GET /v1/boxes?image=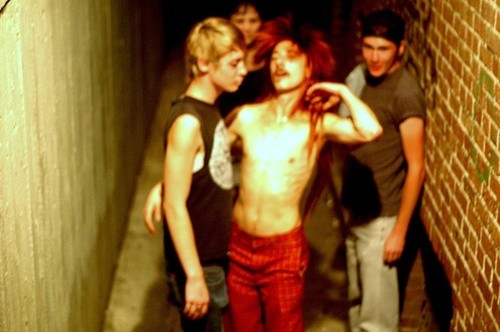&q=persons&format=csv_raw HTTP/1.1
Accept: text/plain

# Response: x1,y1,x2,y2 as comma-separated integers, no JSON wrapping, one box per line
332,8,430,332
214,0,267,119
141,13,384,332
159,17,250,332
